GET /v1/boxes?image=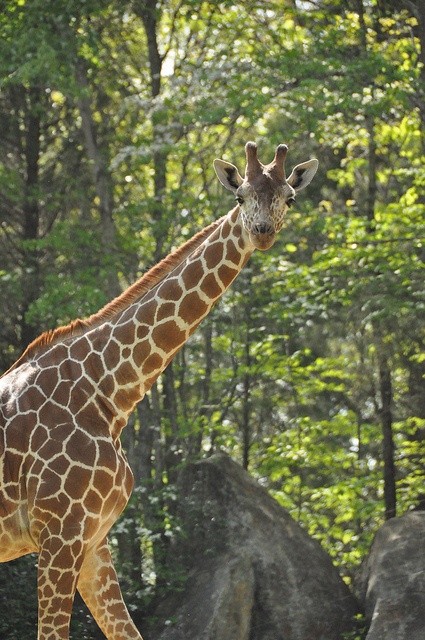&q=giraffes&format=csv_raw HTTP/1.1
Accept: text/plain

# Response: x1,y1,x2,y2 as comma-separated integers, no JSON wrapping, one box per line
1,139,321,640
